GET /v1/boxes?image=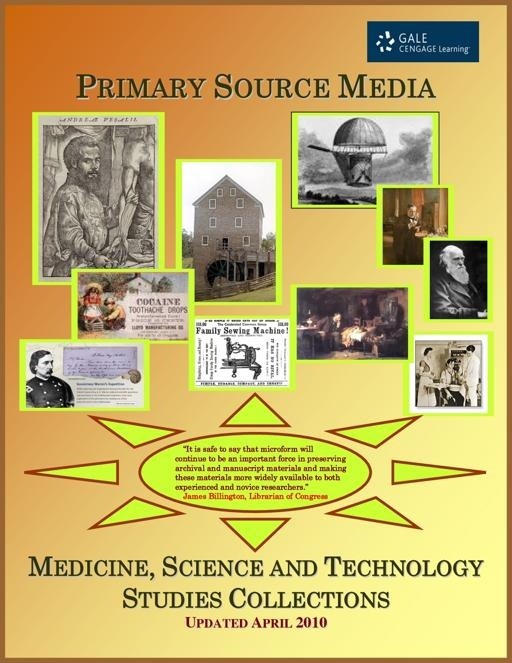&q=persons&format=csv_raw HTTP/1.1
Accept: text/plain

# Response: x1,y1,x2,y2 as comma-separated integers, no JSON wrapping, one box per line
80,282,126,332
430,245,488,318
416,344,481,407
26,350,75,408
392,204,418,264
43,135,120,276
109,124,155,265
315,313,342,352
